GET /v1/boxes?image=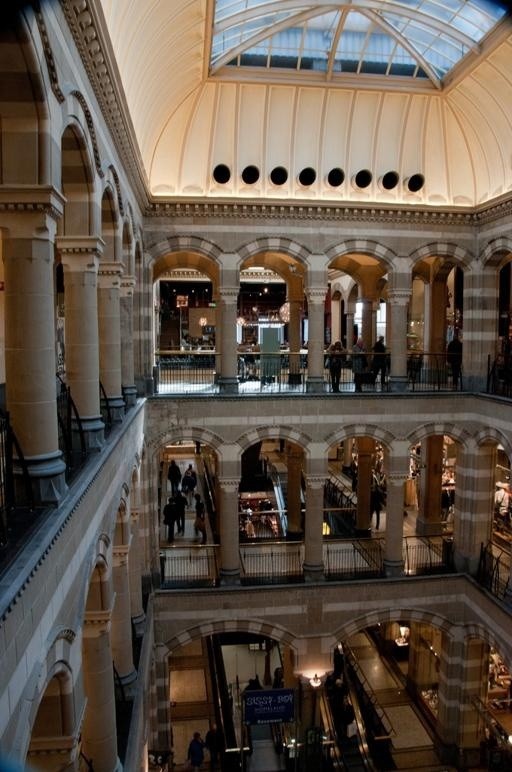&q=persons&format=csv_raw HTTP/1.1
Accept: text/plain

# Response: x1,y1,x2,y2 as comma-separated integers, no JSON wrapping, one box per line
351,339,368,392
161,457,209,546
327,341,348,393
187,732,205,771
370,335,388,390
205,721,225,772
